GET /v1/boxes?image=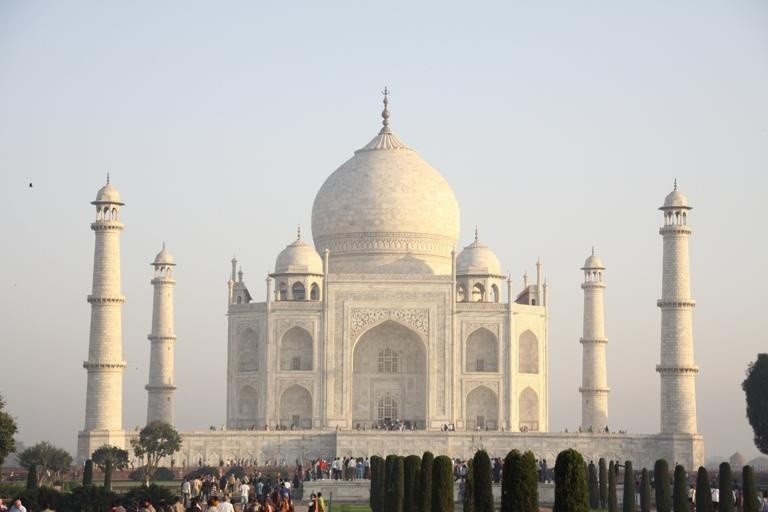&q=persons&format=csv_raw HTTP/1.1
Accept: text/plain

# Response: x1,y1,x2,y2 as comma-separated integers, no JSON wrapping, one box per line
355,416,419,432
687,484,767,512
485,425,488,432
0,489,295,512
170,453,370,490
558,423,627,434
208,421,297,433
314,493,326,512
473,425,482,432
307,494,317,512
501,426,504,432
613,460,624,482
587,460,594,477
451,453,549,484
519,424,528,433
439,423,454,432
334,423,339,431
